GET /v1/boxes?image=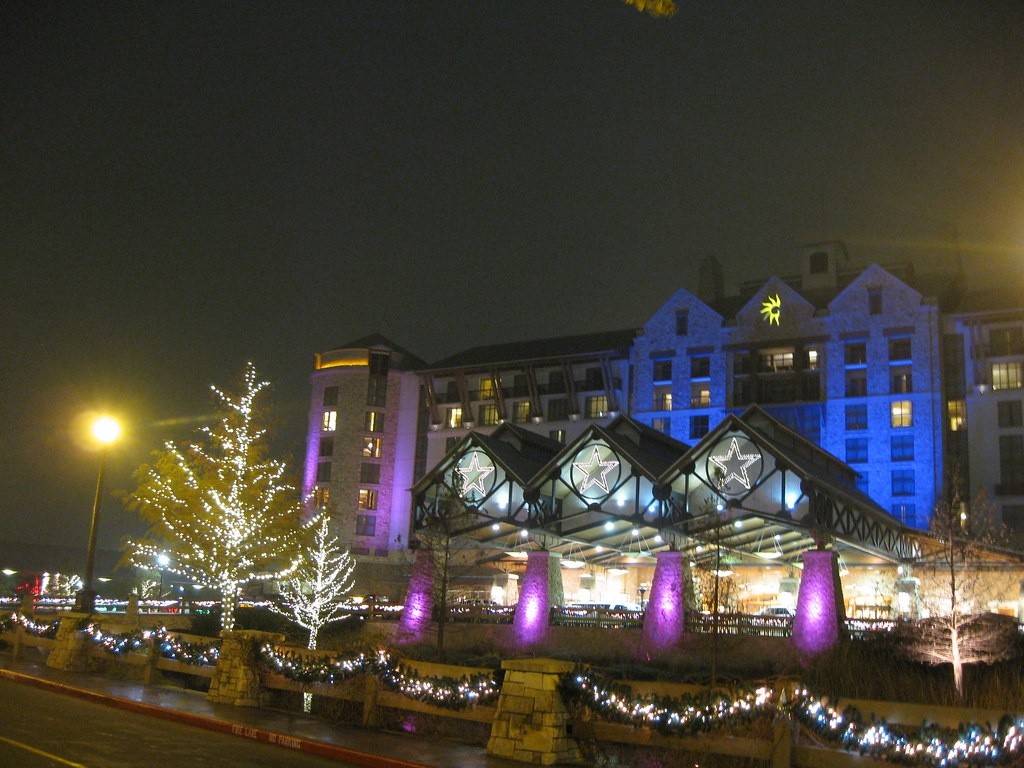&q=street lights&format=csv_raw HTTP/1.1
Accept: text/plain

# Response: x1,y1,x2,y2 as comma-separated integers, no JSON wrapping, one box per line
68,412,119,613
157,554,170,613
